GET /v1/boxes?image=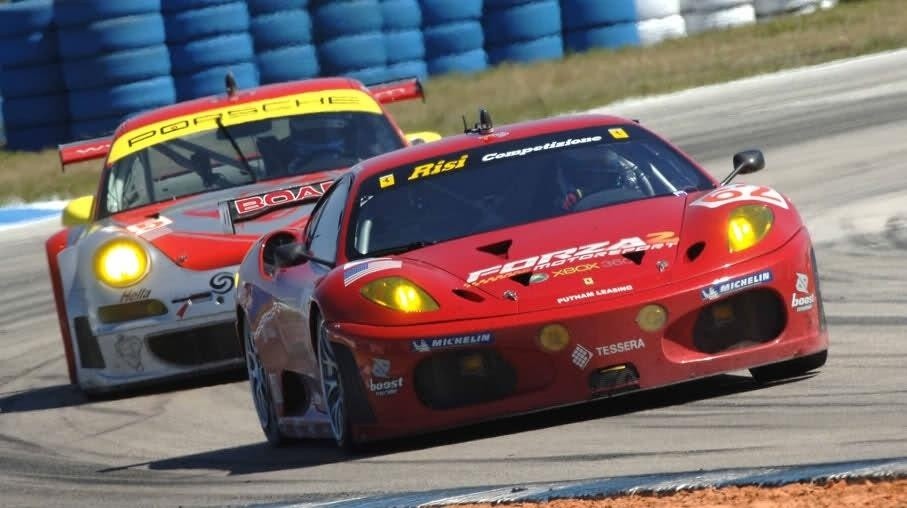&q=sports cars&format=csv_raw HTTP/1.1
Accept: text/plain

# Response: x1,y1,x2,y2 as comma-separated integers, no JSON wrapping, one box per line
234,108,826,459
42,70,440,400
